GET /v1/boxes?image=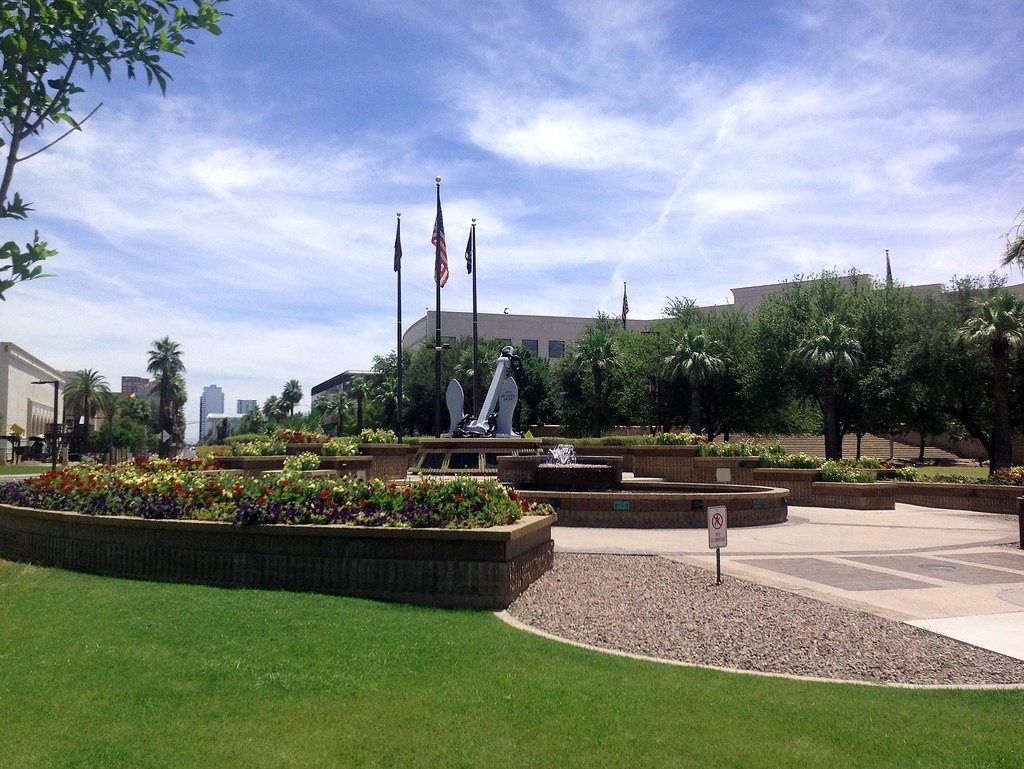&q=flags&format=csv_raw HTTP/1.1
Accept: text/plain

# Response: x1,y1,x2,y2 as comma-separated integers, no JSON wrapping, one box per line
431,202,449,288
622,296,629,324
394,222,402,272
465,227,472,274
